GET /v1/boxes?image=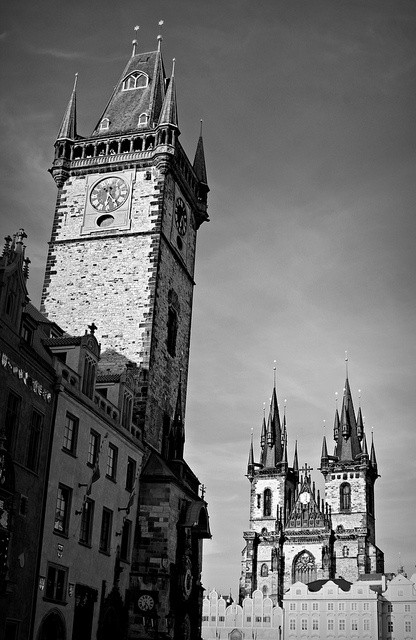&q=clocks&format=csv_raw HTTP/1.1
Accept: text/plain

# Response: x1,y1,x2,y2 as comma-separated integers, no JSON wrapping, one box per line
174,197,187,236
299,492,311,505
89,176,129,213
